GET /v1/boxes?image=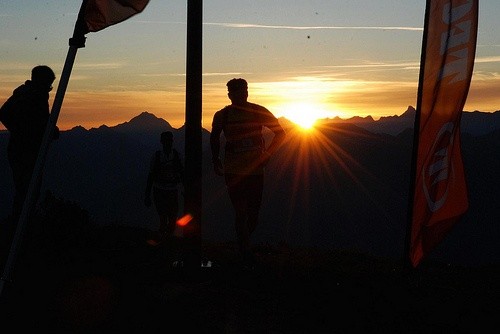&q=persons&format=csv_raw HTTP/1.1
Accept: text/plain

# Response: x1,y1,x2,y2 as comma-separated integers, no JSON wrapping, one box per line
144,131,185,253
0,64,62,230
210,77,283,280
